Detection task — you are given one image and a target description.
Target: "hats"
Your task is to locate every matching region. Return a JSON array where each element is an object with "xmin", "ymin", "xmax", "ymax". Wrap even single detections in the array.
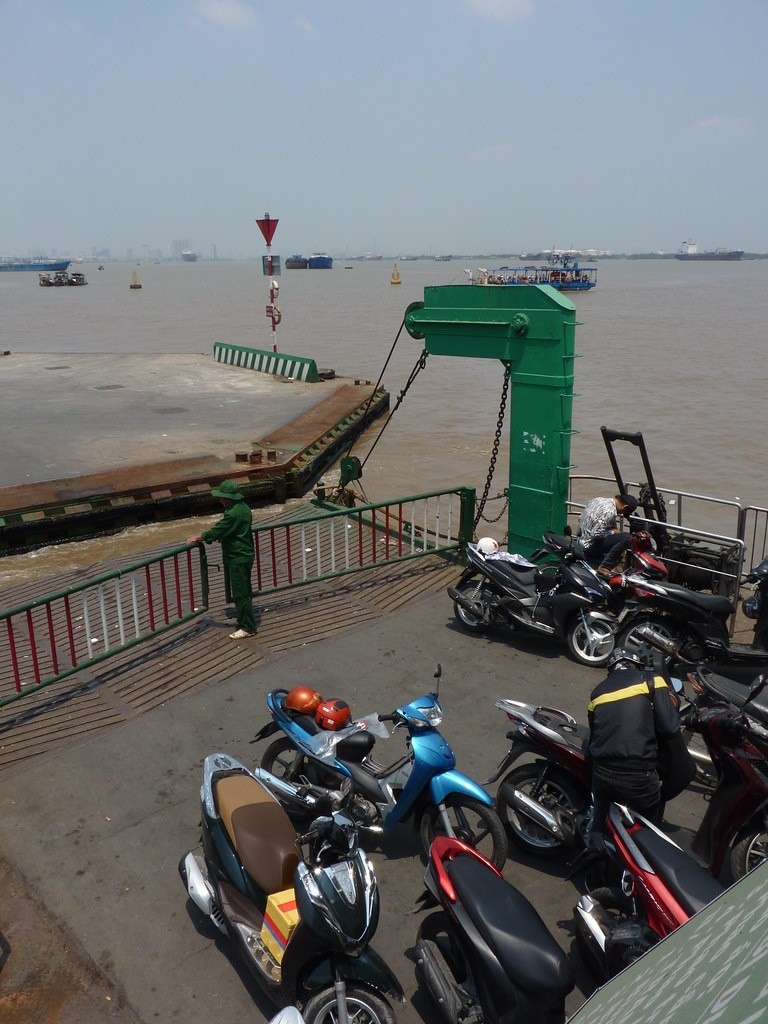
[
  {"xmin": 622, "ymin": 495, "xmax": 638, "ymax": 519},
  {"xmin": 210, "ymin": 480, "xmax": 244, "ymax": 500}
]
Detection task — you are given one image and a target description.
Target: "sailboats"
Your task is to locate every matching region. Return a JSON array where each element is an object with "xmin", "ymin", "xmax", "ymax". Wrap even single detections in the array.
[{"xmin": 129, "ymin": 270, "xmax": 142, "ymax": 289}]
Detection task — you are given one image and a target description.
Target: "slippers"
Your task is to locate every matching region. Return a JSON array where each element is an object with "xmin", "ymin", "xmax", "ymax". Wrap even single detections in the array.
[{"xmin": 596, "ymin": 571, "xmax": 617, "ymax": 580}]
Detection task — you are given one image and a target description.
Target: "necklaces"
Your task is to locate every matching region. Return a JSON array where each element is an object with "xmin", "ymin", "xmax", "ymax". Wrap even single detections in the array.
[{"xmin": 576, "ymin": 494, "xmax": 638, "ymax": 579}]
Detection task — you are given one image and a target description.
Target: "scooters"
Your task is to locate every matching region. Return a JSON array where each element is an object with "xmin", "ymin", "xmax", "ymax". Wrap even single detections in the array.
[
  {"xmin": 177, "ymin": 752, "xmax": 407, "ymax": 1024},
  {"xmin": 404, "ymin": 835, "xmax": 662, "ymax": 1024},
  {"xmin": 563, "ymin": 800, "xmax": 727, "ymax": 991},
  {"xmin": 542, "ymin": 524, "xmax": 768, "ymax": 789},
  {"xmin": 478, "ymin": 671, "xmax": 768, "ymax": 885}
]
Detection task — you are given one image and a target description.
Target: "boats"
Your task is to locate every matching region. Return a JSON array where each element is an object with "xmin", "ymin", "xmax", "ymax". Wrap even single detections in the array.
[
  {"xmin": 180, "ymin": 246, "xmax": 199, "ymax": 263},
  {"xmin": 434, "ymin": 253, "xmax": 453, "ymax": 262},
  {"xmin": 308, "ymin": 251, "xmax": 334, "ymax": 270},
  {"xmin": 284, "ymin": 251, "xmax": 309, "ymax": 269},
  {"xmin": 0, "ymin": 258, "xmax": 72, "ymax": 273},
  {"xmin": 97, "ymin": 264, "xmax": 104, "ymax": 271},
  {"xmin": 674, "ymin": 236, "xmax": 744, "ymax": 262},
  {"xmin": 39, "ymin": 268, "xmax": 88, "ymax": 287},
  {"xmin": 461, "ymin": 240, "xmax": 600, "ymax": 291},
  {"xmin": 389, "ymin": 261, "xmax": 403, "ymax": 284}
]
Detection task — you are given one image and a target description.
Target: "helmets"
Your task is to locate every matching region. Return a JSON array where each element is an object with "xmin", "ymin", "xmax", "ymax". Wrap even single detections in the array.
[
  {"xmin": 476, "ymin": 537, "xmax": 499, "ymax": 555},
  {"xmin": 315, "ymin": 697, "xmax": 352, "ymax": 731},
  {"xmin": 281, "ymin": 683, "xmax": 323, "ymax": 717},
  {"xmin": 607, "ymin": 646, "xmax": 644, "ymax": 668}
]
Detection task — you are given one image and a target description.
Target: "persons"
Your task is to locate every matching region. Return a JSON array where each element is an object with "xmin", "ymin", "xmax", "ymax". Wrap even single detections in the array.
[
  {"xmin": 487, "ymin": 273, "xmax": 590, "ymax": 284},
  {"xmin": 587, "ymin": 660, "xmax": 682, "ymax": 839},
  {"xmin": 185, "ymin": 480, "xmax": 257, "ymax": 639}
]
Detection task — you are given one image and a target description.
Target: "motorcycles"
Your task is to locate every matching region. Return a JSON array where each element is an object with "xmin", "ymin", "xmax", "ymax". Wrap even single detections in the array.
[
  {"xmin": 249, "ymin": 664, "xmax": 509, "ymax": 874},
  {"xmin": 447, "ymin": 525, "xmax": 625, "ymax": 668}
]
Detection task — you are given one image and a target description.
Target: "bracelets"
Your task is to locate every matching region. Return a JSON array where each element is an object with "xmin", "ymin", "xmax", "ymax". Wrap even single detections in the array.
[{"xmin": 611, "ymin": 530, "xmax": 615, "ymax": 535}]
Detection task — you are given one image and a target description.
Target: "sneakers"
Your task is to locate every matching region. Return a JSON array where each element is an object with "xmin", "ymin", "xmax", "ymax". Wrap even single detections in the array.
[
  {"xmin": 222, "ymin": 618, "xmax": 240, "ymax": 627},
  {"xmin": 229, "ymin": 628, "xmax": 256, "ymax": 640}
]
